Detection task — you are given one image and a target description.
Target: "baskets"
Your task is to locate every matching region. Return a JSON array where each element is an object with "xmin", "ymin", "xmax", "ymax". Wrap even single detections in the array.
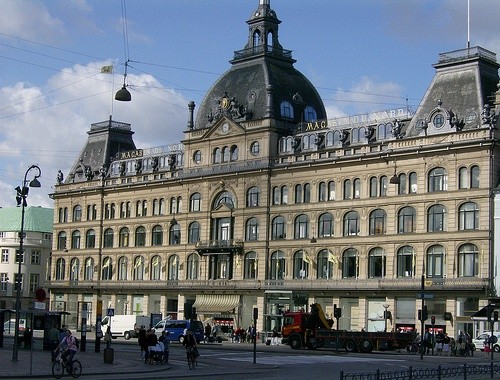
[{"xmin": 51, "ymin": 350, "xmax": 62, "ymax": 363}]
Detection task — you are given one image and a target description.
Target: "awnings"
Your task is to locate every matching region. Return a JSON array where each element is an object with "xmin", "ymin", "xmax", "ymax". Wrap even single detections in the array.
[
  {"xmin": 192, "ymin": 295, "xmax": 240, "ymax": 311},
  {"xmin": 471, "ymin": 303, "xmax": 500, "ymax": 321}
]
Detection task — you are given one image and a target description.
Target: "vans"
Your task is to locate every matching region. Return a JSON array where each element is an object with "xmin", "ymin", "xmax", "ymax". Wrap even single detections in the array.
[
  {"xmin": 471, "ymin": 332, "xmax": 500, "ymax": 352},
  {"xmin": 151, "ymin": 319, "xmax": 204, "ymax": 343},
  {"xmin": 4, "ymin": 318, "xmax": 26, "ymax": 331},
  {"xmin": 100, "ymin": 315, "xmax": 150, "ymax": 340}
]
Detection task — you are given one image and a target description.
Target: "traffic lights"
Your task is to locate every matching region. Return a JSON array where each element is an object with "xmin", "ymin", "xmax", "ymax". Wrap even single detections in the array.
[{"xmin": 431, "ymin": 316, "xmax": 435, "ymax": 324}]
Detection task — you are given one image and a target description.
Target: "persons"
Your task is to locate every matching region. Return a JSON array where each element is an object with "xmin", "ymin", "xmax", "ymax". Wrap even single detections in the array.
[
  {"xmin": 105, "ymin": 326, "xmax": 112, "ymax": 348},
  {"xmin": 272, "ymin": 326, "xmax": 278, "ymax": 345},
  {"xmin": 483, "ymin": 338, "xmax": 489, "ymax": 358},
  {"xmin": 183, "ymin": 330, "xmax": 199, "ymax": 366},
  {"xmin": 396, "ymin": 328, "xmax": 473, "ymax": 357},
  {"xmin": 57, "ymin": 330, "xmax": 77, "ymax": 374},
  {"xmin": 204, "ymin": 322, "xmax": 218, "ymax": 337},
  {"xmin": 138, "ymin": 325, "xmax": 169, "ymax": 364},
  {"xmin": 49, "ymin": 325, "xmax": 67, "ymax": 362},
  {"xmin": 231, "ymin": 326, "xmax": 254, "ymax": 343}
]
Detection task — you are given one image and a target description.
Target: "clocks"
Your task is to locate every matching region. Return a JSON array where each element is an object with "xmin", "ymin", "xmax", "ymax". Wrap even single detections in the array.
[{"xmin": 222, "ymin": 122, "xmax": 229, "ymax": 133}]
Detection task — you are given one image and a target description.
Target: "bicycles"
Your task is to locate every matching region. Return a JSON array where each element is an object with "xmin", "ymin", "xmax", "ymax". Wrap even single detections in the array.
[
  {"xmin": 463, "ymin": 343, "xmax": 474, "ymax": 357},
  {"xmin": 184, "ymin": 345, "xmax": 200, "ymax": 371},
  {"xmin": 51, "ymin": 347, "xmax": 83, "ymax": 380}
]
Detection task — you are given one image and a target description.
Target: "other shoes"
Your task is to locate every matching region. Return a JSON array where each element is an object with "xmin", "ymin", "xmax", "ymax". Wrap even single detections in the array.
[{"xmin": 70, "ymin": 367, "xmax": 73, "ymax": 372}]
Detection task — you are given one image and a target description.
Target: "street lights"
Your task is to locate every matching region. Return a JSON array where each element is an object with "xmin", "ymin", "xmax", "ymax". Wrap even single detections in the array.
[
  {"xmin": 12, "ymin": 165, "xmax": 41, "ymax": 361},
  {"xmin": 383, "ymin": 304, "xmax": 391, "ymax": 332}
]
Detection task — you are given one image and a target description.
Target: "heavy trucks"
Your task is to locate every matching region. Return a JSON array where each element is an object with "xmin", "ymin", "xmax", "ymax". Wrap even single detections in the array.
[{"xmin": 280, "ymin": 303, "xmax": 415, "ymax": 354}]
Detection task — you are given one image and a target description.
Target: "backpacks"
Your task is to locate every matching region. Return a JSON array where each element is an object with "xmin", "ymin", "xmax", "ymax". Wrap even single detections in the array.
[
  {"xmin": 70, "ymin": 336, "xmax": 79, "ymax": 349},
  {"xmin": 105, "ymin": 332, "xmax": 110, "ymax": 341}
]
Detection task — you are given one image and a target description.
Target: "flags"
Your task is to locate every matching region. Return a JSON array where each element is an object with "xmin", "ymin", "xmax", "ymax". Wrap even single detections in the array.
[
  {"xmin": 152, "ymin": 258, "xmax": 158, "ymax": 266},
  {"xmin": 303, "ymin": 253, "xmax": 314, "ymax": 268},
  {"xmin": 103, "ymin": 260, "xmax": 109, "ymax": 268},
  {"xmin": 134, "ymin": 257, "xmax": 141, "ymax": 268},
  {"xmin": 173, "ymin": 259, "xmax": 176, "ymax": 265},
  {"xmin": 72, "ymin": 259, "xmax": 90, "ymax": 272},
  {"xmin": 328, "ymin": 252, "xmax": 341, "ymax": 268},
  {"xmin": 236, "ymin": 256, "xmax": 279, "ymax": 269}
]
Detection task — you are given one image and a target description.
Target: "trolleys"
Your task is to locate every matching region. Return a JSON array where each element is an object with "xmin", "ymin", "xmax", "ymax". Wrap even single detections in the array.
[{"xmin": 148, "ymin": 344, "xmax": 169, "ymax": 366}]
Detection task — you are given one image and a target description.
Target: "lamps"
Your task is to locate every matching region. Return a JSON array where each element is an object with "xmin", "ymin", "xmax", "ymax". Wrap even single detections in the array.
[{"xmin": 114, "ymin": 61, "xmax": 131, "ymax": 102}]
